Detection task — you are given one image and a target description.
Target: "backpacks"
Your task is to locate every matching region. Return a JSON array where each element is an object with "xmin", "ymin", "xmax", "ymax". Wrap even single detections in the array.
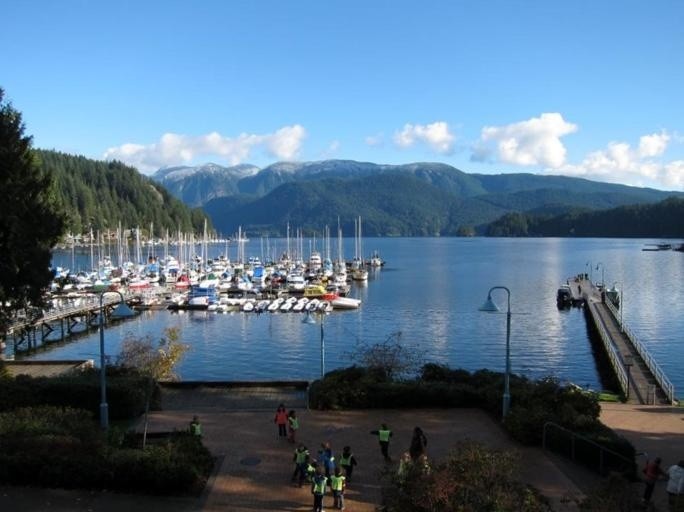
[
  {"xmin": 314, "ymin": 483, "xmax": 325, "ymax": 495},
  {"xmin": 293, "ymin": 421, "xmax": 298, "ymax": 429}
]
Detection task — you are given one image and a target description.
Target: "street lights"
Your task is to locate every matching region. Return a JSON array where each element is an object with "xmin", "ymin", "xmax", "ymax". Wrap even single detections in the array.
[
  {"xmin": 299, "ymin": 305, "xmax": 326, "ymax": 380},
  {"xmin": 585, "ymin": 260, "xmax": 593, "ymax": 281},
  {"xmin": 612, "ymin": 282, "xmax": 624, "ymax": 331},
  {"xmin": 596, "ymin": 260, "xmax": 606, "ymax": 300},
  {"xmin": 97, "ymin": 289, "xmax": 136, "ymax": 433},
  {"xmin": 477, "ymin": 285, "xmax": 513, "ymax": 424}
]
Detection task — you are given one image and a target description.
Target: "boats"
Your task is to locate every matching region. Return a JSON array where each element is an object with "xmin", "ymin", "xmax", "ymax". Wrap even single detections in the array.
[
  {"xmin": 596, "ymin": 280, "xmax": 619, "ymax": 305},
  {"xmin": 556, "ymin": 284, "xmax": 572, "ymax": 303},
  {"xmin": 657, "ymin": 242, "xmax": 671, "ymax": 250}
]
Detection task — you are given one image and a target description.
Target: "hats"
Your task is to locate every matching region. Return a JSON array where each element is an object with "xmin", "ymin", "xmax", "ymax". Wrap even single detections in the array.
[
  {"xmin": 400, "ymin": 448, "xmax": 408, "ymax": 453},
  {"xmin": 296, "ymin": 443, "xmax": 303, "ymax": 447}
]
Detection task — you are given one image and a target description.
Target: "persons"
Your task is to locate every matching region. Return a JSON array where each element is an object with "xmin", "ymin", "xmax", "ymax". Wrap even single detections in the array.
[
  {"xmin": 657, "ymin": 459, "xmax": 684, "ymax": 511},
  {"xmin": 291, "ymin": 441, "xmax": 357, "ymax": 488},
  {"xmin": 640, "ymin": 456, "xmax": 669, "ymax": 501},
  {"xmin": 409, "ymin": 427, "xmax": 427, "ymax": 461},
  {"xmin": 371, "ymin": 423, "xmax": 394, "ymax": 462},
  {"xmin": 188, "ymin": 415, "xmax": 200, "ymax": 437},
  {"xmin": 287, "ymin": 410, "xmax": 298, "ymax": 443},
  {"xmin": 311, "ymin": 466, "xmax": 328, "ymax": 512},
  {"xmin": 329, "ymin": 466, "xmax": 346, "ymax": 510},
  {"xmin": 274, "ymin": 404, "xmax": 289, "ymax": 439}
]
